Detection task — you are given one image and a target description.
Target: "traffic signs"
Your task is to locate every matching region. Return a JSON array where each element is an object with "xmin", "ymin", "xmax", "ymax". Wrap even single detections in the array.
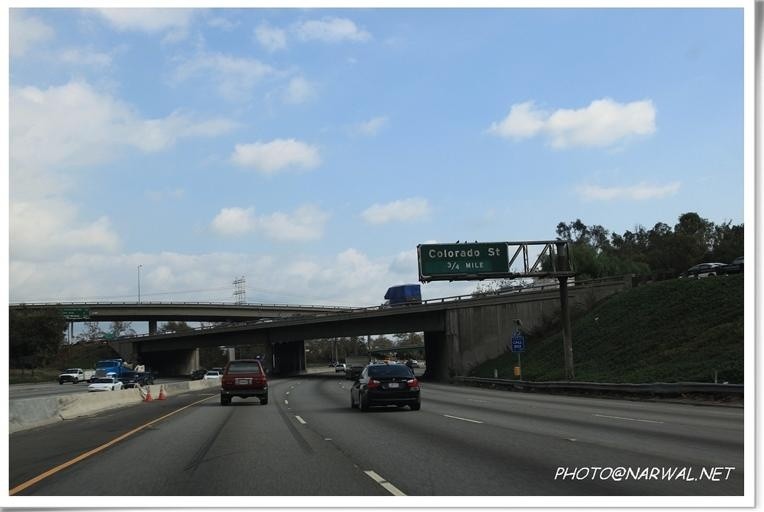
[{"xmin": 417, "ymin": 241, "xmax": 509, "ymax": 279}]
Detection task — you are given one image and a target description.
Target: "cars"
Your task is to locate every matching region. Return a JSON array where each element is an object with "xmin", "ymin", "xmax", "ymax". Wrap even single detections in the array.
[
  {"xmin": 328, "ymin": 357, "xmax": 424, "ymax": 378},
  {"xmin": 729, "ymin": 256, "xmax": 743, "ymax": 274},
  {"xmin": 348, "ymin": 361, "xmax": 422, "ymax": 410},
  {"xmin": 677, "ymin": 261, "xmax": 729, "ymax": 279},
  {"xmin": 58, "ymin": 357, "xmax": 224, "ymax": 391}
]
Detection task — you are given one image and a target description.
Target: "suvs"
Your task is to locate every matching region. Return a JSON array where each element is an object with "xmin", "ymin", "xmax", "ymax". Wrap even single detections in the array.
[{"xmin": 220, "ymin": 358, "xmax": 268, "ymax": 406}]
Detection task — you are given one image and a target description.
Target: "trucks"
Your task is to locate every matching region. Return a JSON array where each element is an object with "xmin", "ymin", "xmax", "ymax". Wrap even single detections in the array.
[{"xmin": 379, "ymin": 284, "xmax": 421, "ymax": 309}]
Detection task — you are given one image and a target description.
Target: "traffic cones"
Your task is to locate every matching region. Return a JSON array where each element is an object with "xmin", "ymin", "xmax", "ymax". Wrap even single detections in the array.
[
  {"xmin": 157, "ymin": 385, "xmax": 168, "ymax": 399},
  {"xmin": 143, "ymin": 385, "xmax": 154, "ymax": 401}
]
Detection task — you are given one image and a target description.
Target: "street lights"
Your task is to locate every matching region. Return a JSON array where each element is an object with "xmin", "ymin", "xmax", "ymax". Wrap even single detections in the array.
[{"xmin": 136, "ymin": 264, "xmax": 144, "ymax": 302}]
